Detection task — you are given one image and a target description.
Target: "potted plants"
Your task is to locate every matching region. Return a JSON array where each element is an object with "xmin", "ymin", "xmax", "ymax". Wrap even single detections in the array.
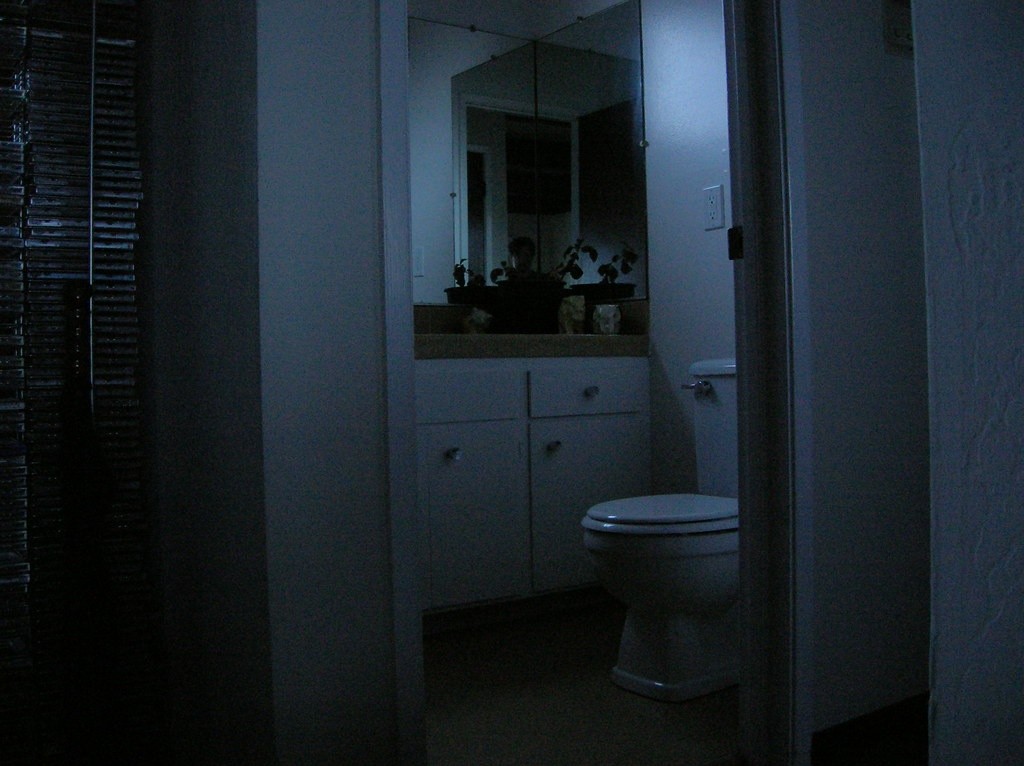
[
  {"xmin": 489, "ymin": 236, "xmax": 597, "ymax": 334},
  {"xmin": 570, "ymin": 240, "xmax": 641, "ymax": 299},
  {"xmin": 443, "ymin": 259, "xmax": 490, "ymax": 303}
]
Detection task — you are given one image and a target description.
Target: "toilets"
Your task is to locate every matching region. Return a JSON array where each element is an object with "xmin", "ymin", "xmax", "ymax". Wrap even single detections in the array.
[{"xmin": 580, "ymin": 355, "xmax": 744, "ymax": 705}]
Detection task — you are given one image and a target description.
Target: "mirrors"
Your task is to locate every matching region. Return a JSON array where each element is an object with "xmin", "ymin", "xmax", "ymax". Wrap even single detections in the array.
[{"xmin": 408, "ymin": 17, "xmax": 649, "ymax": 305}]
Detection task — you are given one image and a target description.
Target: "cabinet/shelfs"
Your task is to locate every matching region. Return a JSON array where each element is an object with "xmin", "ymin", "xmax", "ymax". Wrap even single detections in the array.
[{"xmin": 415, "ymin": 357, "xmax": 649, "ymax": 617}]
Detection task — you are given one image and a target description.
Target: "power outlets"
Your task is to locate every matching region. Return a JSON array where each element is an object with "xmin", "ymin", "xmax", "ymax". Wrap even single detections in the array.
[{"xmin": 701, "ymin": 184, "xmax": 725, "ymax": 231}]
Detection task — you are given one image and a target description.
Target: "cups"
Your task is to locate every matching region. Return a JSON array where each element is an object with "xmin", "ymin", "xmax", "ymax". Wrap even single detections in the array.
[{"xmin": 593, "ymin": 303, "xmax": 622, "ymax": 334}]
